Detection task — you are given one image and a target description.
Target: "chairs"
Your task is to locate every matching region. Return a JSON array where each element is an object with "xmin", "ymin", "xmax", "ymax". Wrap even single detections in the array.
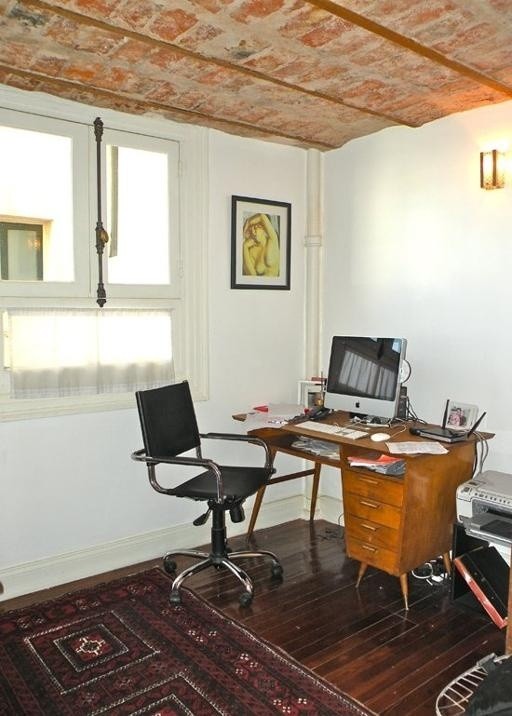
[{"xmin": 130, "ymin": 378, "xmax": 284, "ymax": 608}]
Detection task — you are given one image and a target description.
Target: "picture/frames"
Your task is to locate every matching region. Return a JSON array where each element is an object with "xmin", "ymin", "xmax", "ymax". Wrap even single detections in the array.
[{"xmin": 230, "ymin": 194, "xmax": 292, "ymax": 290}]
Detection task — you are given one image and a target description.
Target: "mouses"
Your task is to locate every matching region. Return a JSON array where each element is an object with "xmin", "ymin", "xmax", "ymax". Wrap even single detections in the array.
[{"xmin": 370, "ymin": 432, "xmax": 391, "ymax": 442}]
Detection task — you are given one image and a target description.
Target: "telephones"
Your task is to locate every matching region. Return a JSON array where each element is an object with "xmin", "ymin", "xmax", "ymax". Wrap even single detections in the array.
[{"xmin": 310, "ymin": 406, "xmax": 330, "ymax": 420}]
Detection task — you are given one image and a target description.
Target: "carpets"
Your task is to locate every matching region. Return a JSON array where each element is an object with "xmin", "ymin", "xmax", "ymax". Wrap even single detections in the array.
[{"xmin": 1, "ymin": 564, "xmax": 377, "ymax": 716}]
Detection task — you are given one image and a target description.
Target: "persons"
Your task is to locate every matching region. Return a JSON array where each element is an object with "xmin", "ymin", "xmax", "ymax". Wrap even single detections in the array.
[
  {"xmin": 447, "ymin": 406, "xmax": 467, "ymax": 427},
  {"xmin": 243, "ymin": 214, "xmax": 279, "ymax": 276}
]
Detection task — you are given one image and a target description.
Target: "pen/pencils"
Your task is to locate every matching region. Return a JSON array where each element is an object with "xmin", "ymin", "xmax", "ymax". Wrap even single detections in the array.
[{"xmin": 367, "ymin": 423, "xmax": 390, "ymax": 428}]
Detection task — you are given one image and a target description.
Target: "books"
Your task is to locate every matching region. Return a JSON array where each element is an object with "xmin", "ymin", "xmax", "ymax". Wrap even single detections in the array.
[
  {"xmin": 453, "ymin": 546, "xmax": 508, "ymax": 630},
  {"xmin": 292, "ymin": 435, "xmax": 450, "ymax": 475},
  {"xmin": 420, "ymin": 426, "xmax": 466, "ymax": 443},
  {"xmin": 461, "ymin": 546, "xmax": 509, "ymax": 620},
  {"xmin": 297, "ymin": 375, "xmax": 329, "ymax": 417}
]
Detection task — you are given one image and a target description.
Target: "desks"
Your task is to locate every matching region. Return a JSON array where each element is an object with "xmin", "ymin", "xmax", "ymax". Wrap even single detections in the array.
[{"xmin": 232, "ymin": 410, "xmax": 495, "ymax": 614}]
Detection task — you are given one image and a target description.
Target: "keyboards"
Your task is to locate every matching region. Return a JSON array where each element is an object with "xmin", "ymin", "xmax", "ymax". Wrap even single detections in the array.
[{"xmin": 292, "ymin": 420, "xmax": 369, "ymax": 441}]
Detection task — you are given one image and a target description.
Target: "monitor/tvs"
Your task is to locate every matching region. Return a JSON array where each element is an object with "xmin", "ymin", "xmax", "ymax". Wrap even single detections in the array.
[{"xmin": 322, "ymin": 336, "xmax": 407, "ymax": 426}]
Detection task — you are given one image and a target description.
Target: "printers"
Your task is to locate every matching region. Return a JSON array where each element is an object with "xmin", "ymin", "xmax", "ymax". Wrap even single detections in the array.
[{"xmin": 456, "ymin": 470, "xmax": 511, "ymax": 549}]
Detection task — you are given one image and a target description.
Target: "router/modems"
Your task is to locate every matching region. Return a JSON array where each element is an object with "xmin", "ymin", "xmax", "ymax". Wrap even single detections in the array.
[{"xmin": 410, "ymin": 400, "xmax": 487, "ymax": 444}]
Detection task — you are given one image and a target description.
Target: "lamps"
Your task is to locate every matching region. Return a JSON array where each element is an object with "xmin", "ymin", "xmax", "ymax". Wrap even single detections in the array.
[{"xmin": 479, "ymin": 148, "xmax": 506, "ymax": 191}]
